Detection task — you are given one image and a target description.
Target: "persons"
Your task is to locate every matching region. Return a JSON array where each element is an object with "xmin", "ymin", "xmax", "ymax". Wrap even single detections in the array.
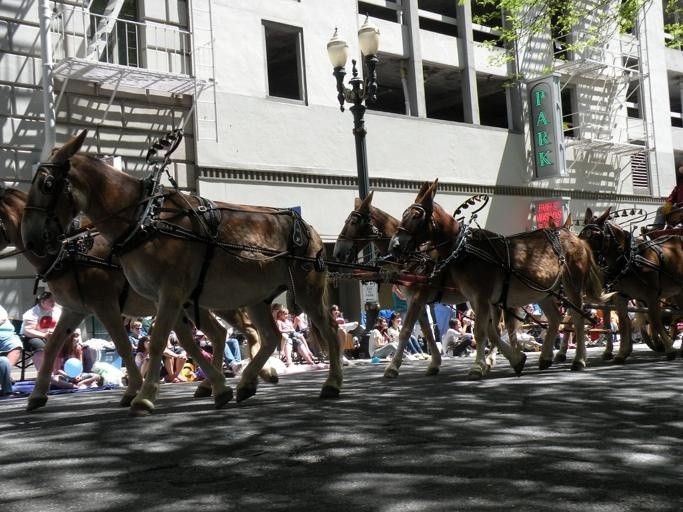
[
  {"xmin": 1, "ymin": 292, "xmax": 359, "ymax": 395},
  {"xmin": 645, "ymin": 179, "xmax": 683, "ymax": 240},
  {"xmin": 516, "ymin": 302, "xmax": 619, "ymax": 350},
  {"xmin": 360, "ymin": 301, "xmax": 476, "ymax": 362}
]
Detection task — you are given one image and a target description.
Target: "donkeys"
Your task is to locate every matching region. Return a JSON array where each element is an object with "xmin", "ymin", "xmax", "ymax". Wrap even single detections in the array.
[
  {"xmin": 386, "ymin": 179, "xmax": 600, "ymax": 380},
  {"xmin": 331, "ymin": 191, "xmax": 528, "ymax": 375},
  {"xmin": 540, "ymin": 209, "xmax": 682, "ymax": 363},
  {"xmin": 0, "ymin": 178, "xmax": 277, "ymax": 410},
  {"xmin": 21, "ymin": 128, "xmax": 344, "ymax": 417}
]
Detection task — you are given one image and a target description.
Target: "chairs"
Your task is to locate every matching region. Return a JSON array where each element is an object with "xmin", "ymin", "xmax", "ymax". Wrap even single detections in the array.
[{"xmin": 0, "ymin": 316, "xmax": 328, "ymax": 397}]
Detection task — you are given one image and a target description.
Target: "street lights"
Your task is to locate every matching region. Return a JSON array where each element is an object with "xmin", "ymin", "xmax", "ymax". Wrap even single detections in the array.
[{"xmin": 325, "ymin": 12, "xmax": 384, "ymax": 358}]
